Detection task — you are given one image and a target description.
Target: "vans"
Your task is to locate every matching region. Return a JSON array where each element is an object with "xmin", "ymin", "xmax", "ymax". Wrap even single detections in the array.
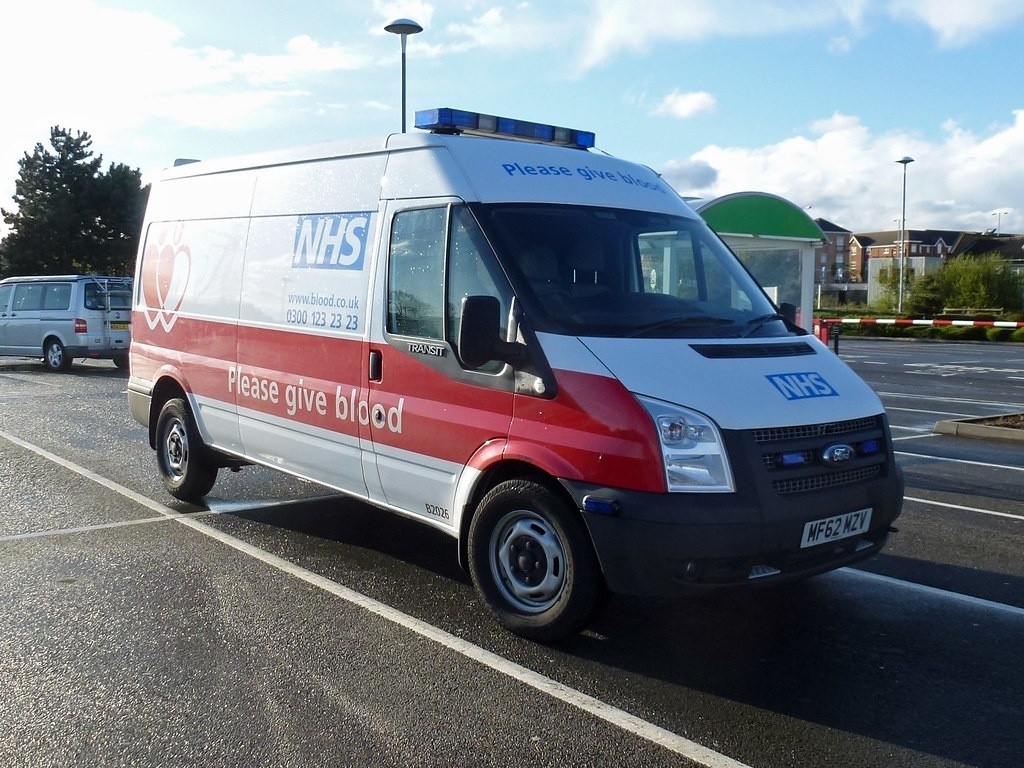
[
  {"xmin": 122, "ymin": 106, "xmax": 907, "ymax": 648},
  {"xmin": 0, "ymin": 276, "xmax": 135, "ymax": 374}
]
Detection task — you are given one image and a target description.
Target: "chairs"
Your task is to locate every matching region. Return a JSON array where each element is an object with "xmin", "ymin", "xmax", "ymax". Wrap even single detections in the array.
[{"xmin": 520, "ymin": 247, "xmax": 570, "ymax": 310}]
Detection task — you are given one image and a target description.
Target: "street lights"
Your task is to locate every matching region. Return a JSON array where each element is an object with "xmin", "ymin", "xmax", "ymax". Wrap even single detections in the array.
[{"xmin": 384, "ymin": 18, "xmax": 423, "ymax": 135}]
